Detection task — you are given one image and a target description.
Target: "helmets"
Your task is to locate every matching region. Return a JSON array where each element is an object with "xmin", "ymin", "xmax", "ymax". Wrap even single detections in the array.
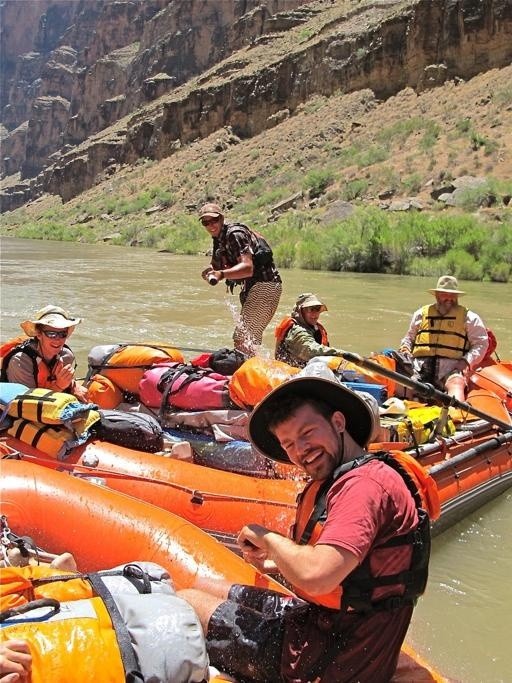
[{"xmin": 245, "ymin": 362, "xmax": 375, "ymax": 467}]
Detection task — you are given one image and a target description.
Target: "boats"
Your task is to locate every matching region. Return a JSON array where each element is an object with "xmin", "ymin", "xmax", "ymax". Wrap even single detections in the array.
[
  {"xmin": 0, "ymin": 449, "xmax": 442, "ymax": 682},
  {"xmin": 0, "ymin": 351, "xmax": 512, "ymax": 563}
]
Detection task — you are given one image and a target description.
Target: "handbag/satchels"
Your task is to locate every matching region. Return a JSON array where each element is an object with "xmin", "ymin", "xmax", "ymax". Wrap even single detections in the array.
[{"xmin": 84, "ymin": 341, "xmax": 301, "ymax": 453}]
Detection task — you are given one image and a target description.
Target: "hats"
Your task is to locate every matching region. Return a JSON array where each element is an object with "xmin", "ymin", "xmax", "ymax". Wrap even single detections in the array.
[
  {"xmin": 20, "ymin": 305, "xmax": 82, "ymax": 339},
  {"xmin": 426, "ymin": 276, "xmax": 467, "ymax": 298},
  {"xmin": 197, "ymin": 203, "xmax": 224, "ymax": 219},
  {"xmin": 297, "ymin": 293, "xmax": 324, "ymax": 309}
]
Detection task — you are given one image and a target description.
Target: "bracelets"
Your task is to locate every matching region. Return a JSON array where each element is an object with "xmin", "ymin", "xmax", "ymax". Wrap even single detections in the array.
[
  {"xmin": 218, "ymin": 269, "xmax": 225, "ymax": 280},
  {"xmin": 55, "ymin": 382, "xmax": 64, "ymax": 391}
]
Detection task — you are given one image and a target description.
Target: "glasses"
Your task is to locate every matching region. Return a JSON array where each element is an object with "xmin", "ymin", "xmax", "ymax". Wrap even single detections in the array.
[
  {"xmin": 201, "ymin": 216, "xmax": 220, "ymax": 226},
  {"xmin": 39, "ymin": 327, "xmax": 68, "ymax": 338},
  {"xmin": 303, "ymin": 306, "xmax": 321, "ymax": 313}
]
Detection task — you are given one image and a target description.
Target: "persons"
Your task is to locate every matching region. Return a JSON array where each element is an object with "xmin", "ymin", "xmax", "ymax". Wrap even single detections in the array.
[
  {"xmin": 398, "ymin": 275, "xmax": 490, "ymax": 402},
  {"xmin": 275, "ymin": 292, "xmax": 365, "ymax": 370},
  {"xmin": 176, "ymin": 361, "xmax": 432, "ymax": 682},
  {"xmin": 0, "ymin": 304, "xmax": 88, "ymax": 404},
  {"xmin": 0, "ymin": 638, "xmax": 34, "ymax": 683},
  {"xmin": 197, "ymin": 203, "xmax": 282, "ymax": 355}
]
